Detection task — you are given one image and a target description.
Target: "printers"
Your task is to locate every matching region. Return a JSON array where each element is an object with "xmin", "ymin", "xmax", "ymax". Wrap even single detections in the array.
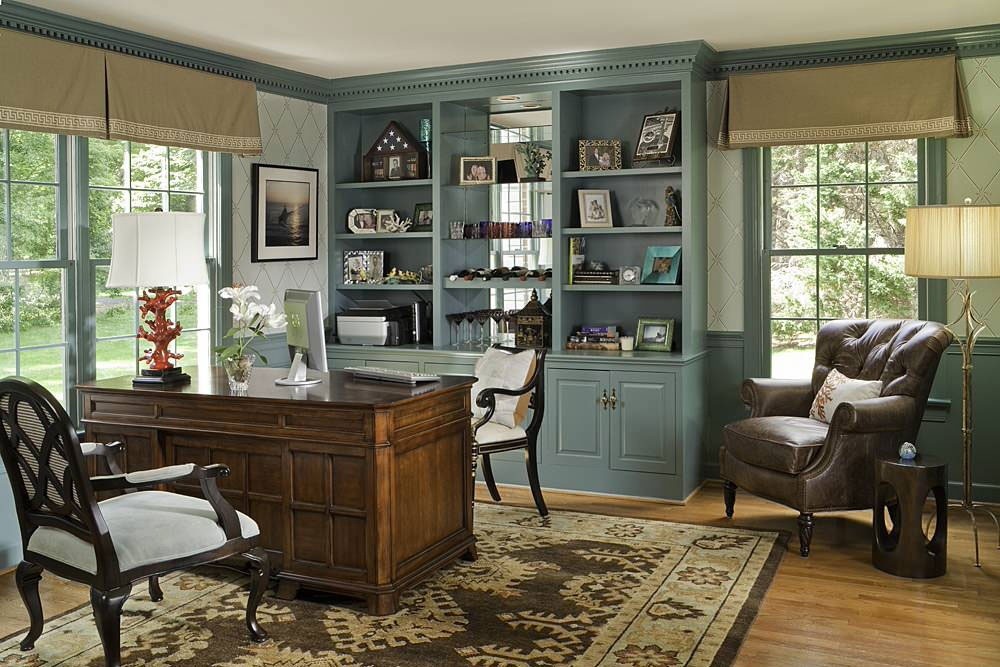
[{"xmin": 333, "ymin": 289, "xmax": 427, "ymax": 347}]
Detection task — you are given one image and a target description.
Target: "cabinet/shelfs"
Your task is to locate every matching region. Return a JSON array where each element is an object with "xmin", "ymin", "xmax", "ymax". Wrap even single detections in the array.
[{"xmin": 325, "ymin": 72, "xmax": 708, "ymax": 507}]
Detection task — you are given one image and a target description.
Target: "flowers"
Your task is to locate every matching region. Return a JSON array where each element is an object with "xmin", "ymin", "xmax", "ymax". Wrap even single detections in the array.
[{"xmin": 215, "ymin": 281, "xmax": 291, "ymax": 367}]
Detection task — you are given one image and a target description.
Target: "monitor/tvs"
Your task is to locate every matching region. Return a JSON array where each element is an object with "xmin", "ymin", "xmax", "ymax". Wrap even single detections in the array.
[{"xmin": 274, "ymin": 288, "xmax": 329, "ymax": 385}]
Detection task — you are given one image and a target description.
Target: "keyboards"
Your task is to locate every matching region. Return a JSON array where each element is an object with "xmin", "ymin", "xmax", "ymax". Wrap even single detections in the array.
[{"xmin": 344, "ymin": 365, "xmax": 441, "ymax": 384}]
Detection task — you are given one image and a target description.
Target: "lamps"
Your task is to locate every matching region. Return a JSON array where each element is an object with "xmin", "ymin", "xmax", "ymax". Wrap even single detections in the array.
[
  {"xmin": 103, "ymin": 208, "xmax": 210, "ymax": 387},
  {"xmin": 902, "ymin": 197, "xmax": 1000, "ymax": 569}
]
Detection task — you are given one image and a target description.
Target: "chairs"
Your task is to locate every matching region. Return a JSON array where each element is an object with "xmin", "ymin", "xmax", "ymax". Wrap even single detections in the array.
[
  {"xmin": 0, "ymin": 374, "xmax": 272, "ymax": 667},
  {"xmin": 720, "ymin": 318, "xmax": 953, "ymax": 555},
  {"xmin": 469, "ymin": 344, "xmax": 550, "ymax": 516}
]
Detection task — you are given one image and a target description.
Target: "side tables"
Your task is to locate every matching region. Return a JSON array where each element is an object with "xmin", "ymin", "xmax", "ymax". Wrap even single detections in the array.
[{"xmin": 872, "ymin": 457, "xmax": 950, "ymax": 578}]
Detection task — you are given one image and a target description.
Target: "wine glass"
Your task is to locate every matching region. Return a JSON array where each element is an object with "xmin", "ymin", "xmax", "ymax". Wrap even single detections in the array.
[{"xmin": 446, "ymin": 309, "xmax": 518, "ymax": 348}]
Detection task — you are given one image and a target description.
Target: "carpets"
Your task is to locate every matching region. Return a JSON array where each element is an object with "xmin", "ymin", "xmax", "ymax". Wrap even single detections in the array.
[{"xmin": 0, "ymin": 499, "xmax": 793, "ymax": 667}]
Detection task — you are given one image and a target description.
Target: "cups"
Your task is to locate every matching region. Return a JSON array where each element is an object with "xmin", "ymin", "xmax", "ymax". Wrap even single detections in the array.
[{"xmin": 450, "ymin": 219, "xmax": 552, "ymax": 239}]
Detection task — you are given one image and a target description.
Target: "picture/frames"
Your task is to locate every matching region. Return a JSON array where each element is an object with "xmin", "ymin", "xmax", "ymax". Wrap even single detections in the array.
[
  {"xmin": 632, "ymin": 111, "xmax": 680, "ymax": 161},
  {"xmin": 576, "ymin": 189, "xmax": 613, "ymax": 228},
  {"xmin": 458, "ymin": 156, "xmax": 498, "ymax": 185},
  {"xmin": 639, "ymin": 245, "xmax": 682, "ymax": 284},
  {"xmin": 411, "ymin": 203, "xmax": 433, "ymax": 230},
  {"xmin": 346, "ymin": 209, "xmax": 400, "ymax": 234},
  {"xmin": 369, "ymin": 250, "xmax": 385, "ymax": 280},
  {"xmin": 579, "ymin": 139, "xmax": 622, "ymax": 170},
  {"xmin": 375, "ymin": 208, "xmax": 395, "ymax": 232},
  {"xmin": 249, "ymin": 162, "xmax": 318, "ymax": 263},
  {"xmin": 342, "ymin": 249, "xmax": 370, "ymax": 283},
  {"xmin": 633, "ymin": 318, "xmax": 675, "ymax": 351}
]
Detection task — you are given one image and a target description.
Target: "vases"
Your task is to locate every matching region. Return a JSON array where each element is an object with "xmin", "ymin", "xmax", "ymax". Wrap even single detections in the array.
[{"xmin": 222, "ymin": 356, "xmax": 254, "ymax": 391}]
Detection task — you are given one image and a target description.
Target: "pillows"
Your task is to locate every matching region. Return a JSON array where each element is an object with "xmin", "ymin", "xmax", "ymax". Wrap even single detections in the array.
[
  {"xmin": 469, "ymin": 348, "xmax": 539, "ymax": 427},
  {"xmin": 805, "ymin": 364, "xmax": 885, "ymax": 426}
]
